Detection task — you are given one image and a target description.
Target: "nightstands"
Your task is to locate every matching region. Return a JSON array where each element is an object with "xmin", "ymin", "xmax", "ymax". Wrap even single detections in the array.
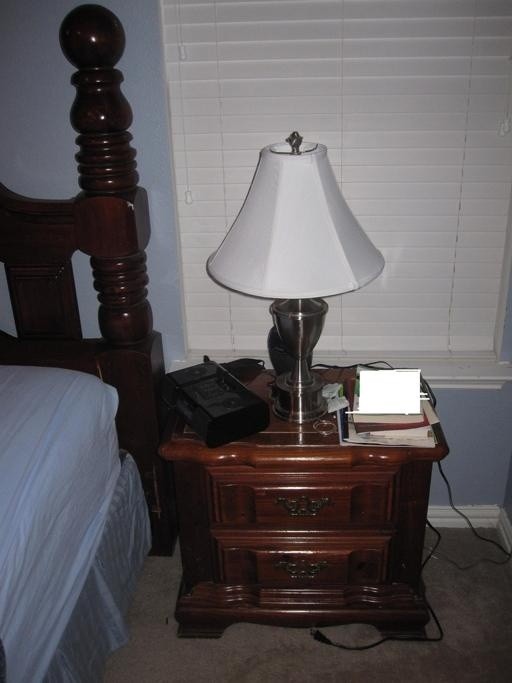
[{"xmin": 156, "ymin": 370, "xmax": 448, "ymax": 644}]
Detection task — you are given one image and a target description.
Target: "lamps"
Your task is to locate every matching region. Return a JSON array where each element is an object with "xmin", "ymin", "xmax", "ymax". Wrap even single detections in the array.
[{"xmin": 204, "ymin": 129, "xmax": 386, "ymax": 424}]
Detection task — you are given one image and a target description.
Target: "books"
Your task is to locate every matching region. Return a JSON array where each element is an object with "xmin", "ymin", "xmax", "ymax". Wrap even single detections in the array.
[{"xmin": 334, "ymin": 363, "xmax": 442, "ymax": 451}]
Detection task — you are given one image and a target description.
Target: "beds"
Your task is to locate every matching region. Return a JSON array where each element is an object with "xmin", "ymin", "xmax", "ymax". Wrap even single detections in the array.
[{"xmin": 1, "ymin": 4, "xmax": 180, "ymax": 683}]
